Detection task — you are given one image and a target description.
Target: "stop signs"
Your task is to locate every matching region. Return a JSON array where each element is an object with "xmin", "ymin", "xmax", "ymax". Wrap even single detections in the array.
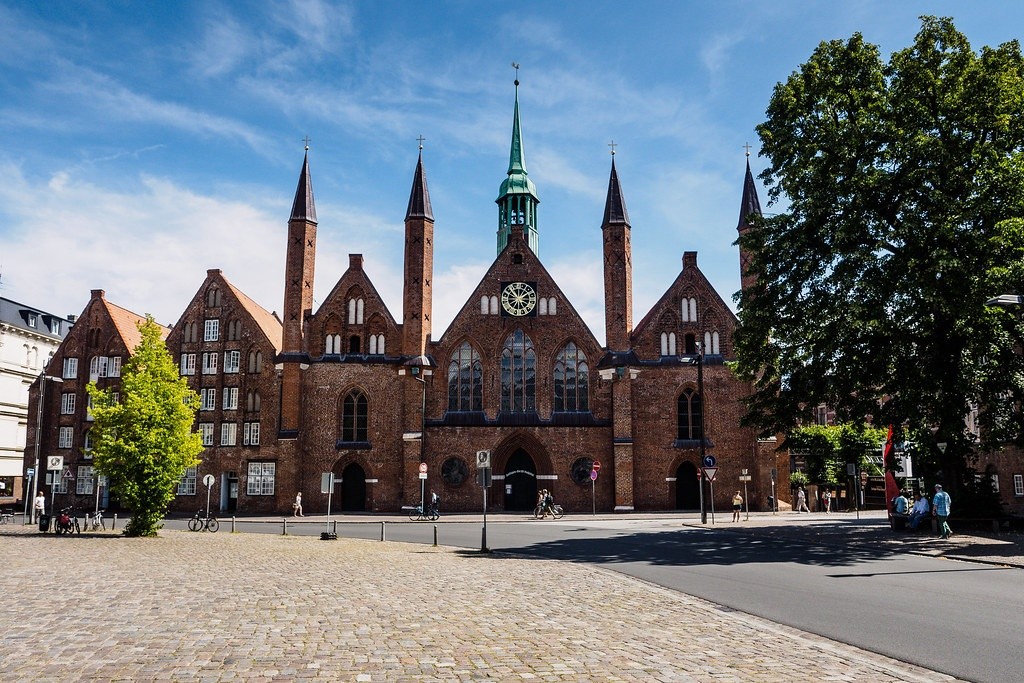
[
  {"xmin": 696, "ymin": 468, "xmax": 701, "ymax": 480},
  {"xmin": 593, "ymin": 461, "xmax": 601, "ymax": 472}
]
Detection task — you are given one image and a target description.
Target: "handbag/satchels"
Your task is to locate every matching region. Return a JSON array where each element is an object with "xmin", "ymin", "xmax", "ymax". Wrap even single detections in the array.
[{"xmin": 292, "ymin": 503, "xmax": 298, "ymax": 508}]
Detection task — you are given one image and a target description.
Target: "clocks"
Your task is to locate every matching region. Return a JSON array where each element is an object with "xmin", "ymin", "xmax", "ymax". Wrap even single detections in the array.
[{"xmin": 501, "ymin": 282, "xmax": 537, "ymax": 317}]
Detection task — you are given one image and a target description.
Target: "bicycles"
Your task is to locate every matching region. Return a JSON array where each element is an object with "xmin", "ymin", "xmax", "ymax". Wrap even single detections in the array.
[
  {"xmin": 535, "ymin": 499, "xmax": 564, "ymax": 520},
  {"xmin": 409, "ymin": 500, "xmax": 440, "ymax": 522},
  {"xmin": 187, "ymin": 509, "xmax": 220, "ymax": 534},
  {"xmin": 70, "ymin": 504, "xmax": 81, "ymax": 535},
  {"xmin": 92, "ymin": 510, "xmax": 106, "ymax": 532},
  {"xmin": 54, "ymin": 505, "xmax": 75, "ymax": 534}
]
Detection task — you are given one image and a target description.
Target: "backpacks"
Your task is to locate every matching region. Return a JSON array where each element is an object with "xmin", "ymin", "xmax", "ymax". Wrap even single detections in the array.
[
  {"xmin": 436, "ymin": 495, "xmax": 441, "ymax": 503},
  {"xmin": 544, "ymin": 495, "xmax": 552, "ymax": 506}
]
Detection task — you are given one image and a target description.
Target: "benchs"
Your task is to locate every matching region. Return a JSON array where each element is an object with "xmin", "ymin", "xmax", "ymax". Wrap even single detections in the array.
[{"xmin": 889, "ymin": 513, "xmax": 999, "ymax": 535}]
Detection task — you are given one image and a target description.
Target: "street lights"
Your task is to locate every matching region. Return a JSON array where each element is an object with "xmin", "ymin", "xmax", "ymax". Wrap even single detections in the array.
[
  {"xmin": 31, "ymin": 370, "xmax": 63, "ymax": 524},
  {"xmin": 681, "ymin": 354, "xmax": 708, "ymax": 525}
]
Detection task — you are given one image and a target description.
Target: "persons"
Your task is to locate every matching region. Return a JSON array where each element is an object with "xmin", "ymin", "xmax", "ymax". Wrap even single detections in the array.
[
  {"xmin": 797, "ymin": 487, "xmax": 810, "ymax": 514},
  {"xmin": 35, "ymin": 491, "xmax": 45, "ymax": 524},
  {"xmin": 430, "ymin": 488, "xmax": 440, "ymax": 520},
  {"xmin": 932, "ymin": 484, "xmax": 953, "ymax": 538},
  {"xmin": 891, "ymin": 491, "xmax": 909, "ymax": 514},
  {"xmin": 541, "ymin": 489, "xmax": 558, "ymax": 519},
  {"xmin": 822, "ymin": 488, "xmax": 832, "ymax": 514},
  {"xmin": 294, "ymin": 491, "xmax": 305, "ymax": 517},
  {"xmin": 732, "ymin": 490, "xmax": 743, "ymax": 522},
  {"xmin": 906, "ymin": 492, "xmax": 930, "ymax": 530},
  {"xmin": 534, "ymin": 490, "xmax": 543, "ymax": 518}
]
